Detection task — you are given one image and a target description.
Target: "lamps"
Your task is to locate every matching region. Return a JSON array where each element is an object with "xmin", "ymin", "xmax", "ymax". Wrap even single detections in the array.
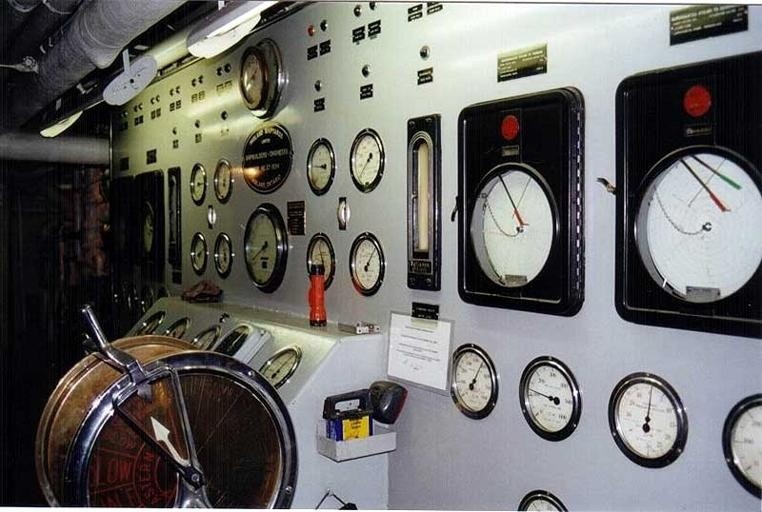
[
  {"xmin": 186, "ymin": 0, "xmax": 279, "ymax": 59},
  {"xmin": 20, "ymin": 82, "xmax": 105, "ymax": 138},
  {"xmin": 102, "ymin": 49, "xmax": 158, "ymax": 106}
]
